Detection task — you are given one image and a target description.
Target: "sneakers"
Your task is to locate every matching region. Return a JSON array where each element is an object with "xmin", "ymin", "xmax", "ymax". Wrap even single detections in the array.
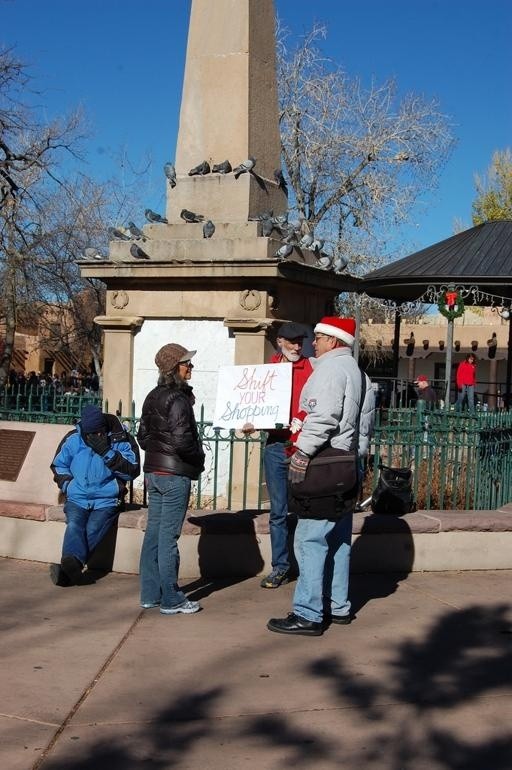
[
  {"xmin": 140, "ymin": 602, "xmax": 161, "ymax": 608},
  {"xmin": 51, "ymin": 564, "xmax": 68, "ymax": 585},
  {"xmin": 260, "ymin": 570, "xmax": 288, "ymax": 588},
  {"xmin": 159, "ymin": 600, "xmax": 200, "ymax": 613},
  {"xmin": 61, "ymin": 556, "xmax": 89, "ymax": 586}
]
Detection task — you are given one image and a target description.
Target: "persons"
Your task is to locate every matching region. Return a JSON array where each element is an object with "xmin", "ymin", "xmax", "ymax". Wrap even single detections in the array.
[
  {"xmin": 243, "ymin": 323, "xmax": 319, "ymax": 589},
  {"xmin": 414, "ymin": 375, "xmax": 439, "ymax": 460},
  {"xmin": 267, "ymin": 316, "xmax": 375, "ymax": 637},
  {"xmin": 48, "ymin": 404, "xmax": 139, "ymax": 586},
  {"xmin": 5, "ymin": 363, "xmax": 98, "ymax": 403},
  {"xmin": 455, "ymin": 353, "xmax": 477, "ymax": 415},
  {"xmin": 136, "ymin": 344, "xmax": 206, "ymax": 615}
]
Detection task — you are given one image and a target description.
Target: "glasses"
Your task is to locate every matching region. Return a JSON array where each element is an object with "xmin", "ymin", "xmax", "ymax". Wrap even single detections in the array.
[{"xmin": 178, "ymin": 360, "xmax": 191, "ymax": 367}]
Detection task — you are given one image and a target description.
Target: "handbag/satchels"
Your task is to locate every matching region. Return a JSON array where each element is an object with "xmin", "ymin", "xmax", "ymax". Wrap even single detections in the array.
[
  {"xmin": 287, "ymin": 448, "xmax": 358, "ymax": 519},
  {"xmin": 371, "ymin": 465, "xmax": 412, "ymax": 516}
]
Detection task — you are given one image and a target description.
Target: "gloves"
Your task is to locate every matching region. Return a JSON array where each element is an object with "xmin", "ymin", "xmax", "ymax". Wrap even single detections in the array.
[
  {"xmin": 282, "ymin": 451, "xmax": 310, "ymax": 484},
  {"xmin": 85, "ymin": 431, "xmax": 110, "ymax": 456}
]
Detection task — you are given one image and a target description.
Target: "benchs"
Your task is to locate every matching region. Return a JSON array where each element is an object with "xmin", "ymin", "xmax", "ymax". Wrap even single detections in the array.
[{"xmin": 0, "ymin": 501, "xmax": 512, "ymax": 581}]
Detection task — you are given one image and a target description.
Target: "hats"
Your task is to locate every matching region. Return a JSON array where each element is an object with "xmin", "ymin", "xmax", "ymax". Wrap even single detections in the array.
[
  {"xmin": 277, "ymin": 323, "xmax": 308, "ymax": 340},
  {"xmin": 81, "ymin": 406, "xmax": 105, "ymax": 433},
  {"xmin": 155, "ymin": 344, "xmax": 197, "ymax": 371},
  {"xmin": 414, "ymin": 375, "xmax": 428, "ymax": 383},
  {"xmin": 314, "ymin": 317, "xmax": 356, "ymax": 347}
]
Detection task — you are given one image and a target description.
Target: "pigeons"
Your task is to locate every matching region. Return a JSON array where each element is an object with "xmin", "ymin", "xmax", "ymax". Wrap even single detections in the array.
[
  {"xmin": 189, "ymin": 157, "xmax": 259, "ymax": 176},
  {"xmin": 130, "ymin": 243, "xmax": 150, "ymax": 260},
  {"xmin": 312, "ymin": 253, "xmax": 335, "ymax": 272},
  {"xmin": 329, "ymin": 252, "xmax": 350, "ymax": 273},
  {"xmin": 255, "ymin": 207, "xmax": 326, "ymax": 259},
  {"xmin": 164, "ymin": 162, "xmax": 175, "ymax": 186},
  {"xmin": 145, "ymin": 209, "xmax": 168, "ymax": 224},
  {"xmin": 77, "ymin": 241, "xmax": 104, "ymax": 260},
  {"xmin": 180, "ymin": 209, "xmax": 204, "ymax": 223},
  {"xmin": 108, "ymin": 222, "xmax": 150, "ymax": 240},
  {"xmin": 273, "ymin": 167, "xmax": 289, "ymax": 186},
  {"xmin": 204, "ymin": 221, "xmax": 215, "ymax": 238}
]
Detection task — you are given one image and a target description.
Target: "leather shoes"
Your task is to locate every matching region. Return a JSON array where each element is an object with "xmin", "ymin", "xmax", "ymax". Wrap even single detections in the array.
[
  {"xmin": 331, "ymin": 614, "xmax": 351, "ymax": 624},
  {"xmin": 268, "ymin": 612, "xmax": 322, "ymax": 635}
]
